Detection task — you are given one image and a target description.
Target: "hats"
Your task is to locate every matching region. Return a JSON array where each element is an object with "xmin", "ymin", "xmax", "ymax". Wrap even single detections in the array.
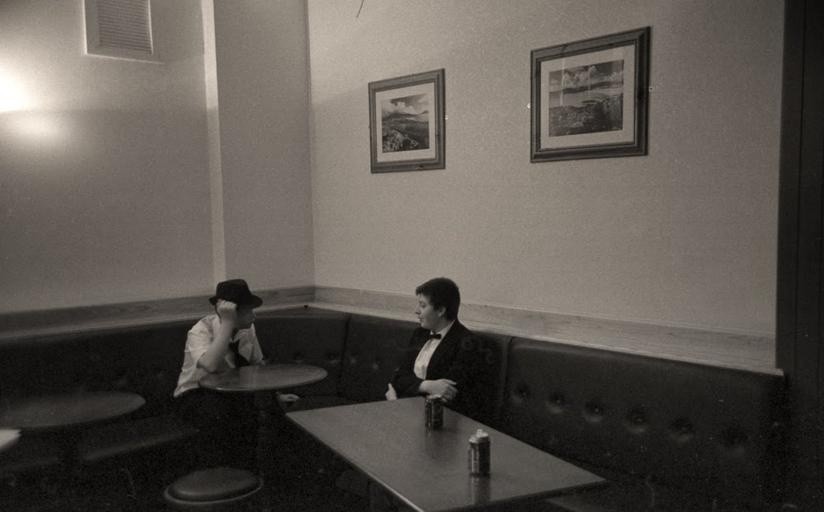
[{"xmin": 209, "ymin": 279, "xmax": 262, "ymax": 308}]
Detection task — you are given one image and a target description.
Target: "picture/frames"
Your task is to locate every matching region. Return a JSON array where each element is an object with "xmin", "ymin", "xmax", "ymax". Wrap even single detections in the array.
[
  {"xmin": 527, "ymin": 23, "xmax": 652, "ymax": 165},
  {"xmin": 365, "ymin": 66, "xmax": 448, "ymax": 175}
]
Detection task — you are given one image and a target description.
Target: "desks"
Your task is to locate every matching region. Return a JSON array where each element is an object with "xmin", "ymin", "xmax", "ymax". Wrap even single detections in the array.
[
  {"xmin": 280, "ymin": 396, "xmax": 609, "ymax": 511},
  {"xmin": 197, "ymin": 360, "xmax": 329, "ymax": 451},
  {"xmin": 0, "ymin": 386, "xmax": 142, "ymax": 447}
]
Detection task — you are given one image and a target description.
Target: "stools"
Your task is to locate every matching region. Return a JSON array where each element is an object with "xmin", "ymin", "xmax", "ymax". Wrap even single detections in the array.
[{"xmin": 160, "ymin": 462, "xmax": 263, "ymax": 510}]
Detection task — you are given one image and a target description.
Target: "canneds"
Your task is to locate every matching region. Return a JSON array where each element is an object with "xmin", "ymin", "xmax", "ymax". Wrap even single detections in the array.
[
  {"xmin": 466, "ymin": 429, "xmax": 491, "ymax": 478},
  {"xmin": 424, "ymin": 394, "xmax": 443, "ymax": 430}
]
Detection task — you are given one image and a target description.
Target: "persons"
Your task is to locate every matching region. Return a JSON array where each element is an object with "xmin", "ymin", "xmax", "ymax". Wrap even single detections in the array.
[
  {"xmin": 378, "ymin": 277, "xmax": 488, "ymax": 423},
  {"xmin": 172, "ymin": 278, "xmax": 299, "ymax": 469}
]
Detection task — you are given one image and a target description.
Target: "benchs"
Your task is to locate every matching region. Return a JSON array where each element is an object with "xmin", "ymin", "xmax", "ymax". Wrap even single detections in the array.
[
  {"xmin": 0, "ymin": 305, "xmax": 795, "ymax": 511},
  {"xmin": 6, "ymin": 406, "xmax": 188, "ymax": 487}
]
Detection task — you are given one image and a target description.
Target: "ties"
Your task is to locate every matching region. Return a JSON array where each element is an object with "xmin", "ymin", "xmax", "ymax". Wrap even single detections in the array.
[{"xmin": 229, "ymin": 340, "xmax": 248, "ymax": 366}]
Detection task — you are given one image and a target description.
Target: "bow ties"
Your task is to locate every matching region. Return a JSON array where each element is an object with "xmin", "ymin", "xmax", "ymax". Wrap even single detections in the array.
[{"xmin": 426, "ymin": 334, "xmax": 441, "ymax": 340}]
